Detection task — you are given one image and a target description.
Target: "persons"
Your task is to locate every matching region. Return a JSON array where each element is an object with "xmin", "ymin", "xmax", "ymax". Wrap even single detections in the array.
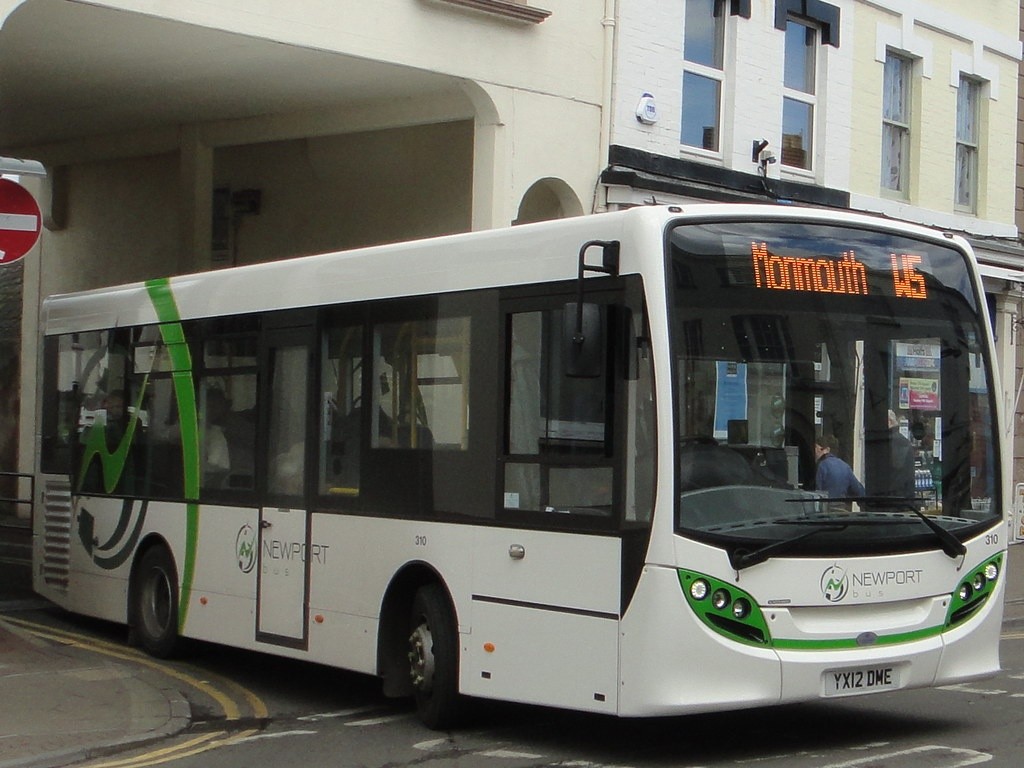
[
  {"xmin": 886, "ymin": 409, "xmax": 916, "ymax": 506},
  {"xmin": 812, "ymin": 434, "xmax": 867, "ymax": 514},
  {"xmin": 68, "ymin": 377, "xmax": 233, "ymax": 484}
]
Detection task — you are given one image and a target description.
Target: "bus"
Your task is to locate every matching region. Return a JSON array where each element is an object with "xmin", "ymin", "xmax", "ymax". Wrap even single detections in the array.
[{"xmin": 31, "ymin": 200, "xmax": 1010, "ymax": 733}]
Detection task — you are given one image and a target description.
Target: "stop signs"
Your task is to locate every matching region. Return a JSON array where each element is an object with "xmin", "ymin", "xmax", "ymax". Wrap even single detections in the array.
[{"xmin": 1, "ymin": 178, "xmax": 42, "ymax": 266}]
